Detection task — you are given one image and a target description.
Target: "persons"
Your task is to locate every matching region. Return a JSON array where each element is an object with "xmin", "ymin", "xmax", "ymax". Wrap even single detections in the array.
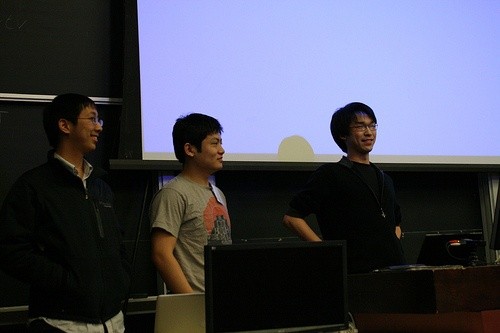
[
  {"xmin": 150, "ymin": 113, "xmax": 236, "ymax": 295},
  {"xmin": 282, "ymin": 102, "xmax": 412, "ymax": 273},
  {"xmin": 1, "ymin": 93, "xmax": 130, "ymax": 333}
]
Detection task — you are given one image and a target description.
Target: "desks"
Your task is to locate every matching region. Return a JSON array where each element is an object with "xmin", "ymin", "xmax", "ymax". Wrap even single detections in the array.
[{"xmin": 350, "ymin": 262, "xmax": 500, "ymax": 332}]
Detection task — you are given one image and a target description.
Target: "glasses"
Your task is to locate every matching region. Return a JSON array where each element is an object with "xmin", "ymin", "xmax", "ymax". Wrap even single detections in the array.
[
  {"xmin": 344, "ymin": 123, "xmax": 378, "ymax": 130},
  {"xmin": 74, "ymin": 116, "xmax": 103, "ymax": 127}
]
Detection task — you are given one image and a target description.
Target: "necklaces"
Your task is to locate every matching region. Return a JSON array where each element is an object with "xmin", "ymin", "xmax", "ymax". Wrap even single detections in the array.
[{"xmin": 342, "ymin": 167, "xmax": 386, "ymax": 219}]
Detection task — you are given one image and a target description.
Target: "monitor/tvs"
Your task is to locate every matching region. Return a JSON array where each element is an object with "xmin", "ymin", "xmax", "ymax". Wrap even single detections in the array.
[
  {"xmin": 203, "ymin": 240, "xmax": 350, "ymax": 333},
  {"xmin": 417, "ymin": 232, "xmax": 483, "ymax": 265}
]
